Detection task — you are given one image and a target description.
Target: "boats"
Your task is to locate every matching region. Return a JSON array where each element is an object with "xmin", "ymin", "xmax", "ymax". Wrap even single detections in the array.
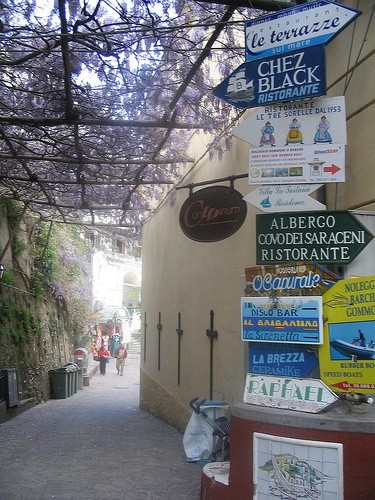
[{"xmin": 329, "ymin": 338, "xmax": 375, "ymax": 359}]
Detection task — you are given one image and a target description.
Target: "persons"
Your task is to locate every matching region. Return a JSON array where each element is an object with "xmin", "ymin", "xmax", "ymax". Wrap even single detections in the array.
[
  {"xmin": 114, "ymin": 344, "xmax": 127, "ymax": 376},
  {"xmin": 110, "ymin": 336, "xmax": 116, "ymax": 357},
  {"xmin": 99, "ymin": 346, "xmax": 109, "ymax": 376}
]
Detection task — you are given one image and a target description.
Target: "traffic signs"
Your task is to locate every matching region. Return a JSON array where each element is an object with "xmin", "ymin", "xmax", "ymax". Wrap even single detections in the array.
[
  {"xmin": 246, "ymin": 341, "xmax": 320, "ymax": 379},
  {"xmin": 240, "ymin": 372, "xmax": 341, "ymax": 410},
  {"xmin": 231, "ymin": 94, "xmax": 347, "ymax": 145},
  {"xmin": 240, "ymin": 298, "xmax": 324, "ymax": 347},
  {"xmin": 210, "ymin": 44, "xmax": 326, "ymax": 112},
  {"xmin": 241, "ymin": 184, "xmax": 328, "ymax": 213},
  {"xmin": 242, "ymin": 4, "xmax": 365, "ymax": 64},
  {"xmin": 319, "ymin": 274, "xmax": 375, "ymax": 393},
  {"xmin": 255, "ymin": 210, "xmax": 375, "ymax": 266},
  {"xmin": 243, "ymin": 260, "xmax": 342, "ymax": 297},
  {"xmin": 248, "ymin": 147, "xmax": 345, "ymax": 184}
]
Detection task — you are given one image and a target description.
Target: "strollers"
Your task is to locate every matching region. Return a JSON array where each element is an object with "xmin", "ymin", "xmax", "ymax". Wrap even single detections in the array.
[{"xmin": 190, "ymin": 395, "xmax": 231, "ymax": 464}]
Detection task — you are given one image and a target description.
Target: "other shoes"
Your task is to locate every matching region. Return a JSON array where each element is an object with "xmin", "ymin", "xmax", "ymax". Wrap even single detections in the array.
[
  {"xmin": 117, "ymin": 371, "xmax": 120, "ymax": 375},
  {"xmin": 120, "ymin": 372, "xmax": 123, "ymax": 376}
]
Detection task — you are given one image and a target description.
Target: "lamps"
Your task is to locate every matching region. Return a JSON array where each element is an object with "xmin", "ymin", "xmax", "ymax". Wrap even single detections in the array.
[{"xmin": 0, "ymin": 264, "xmax": 5, "ymax": 278}]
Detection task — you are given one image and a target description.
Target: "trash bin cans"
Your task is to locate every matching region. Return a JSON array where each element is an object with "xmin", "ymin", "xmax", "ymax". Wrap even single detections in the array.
[
  {"xmin": 50, "ymin": 363, "xmax": 84, "ymax": 399},
  {"xmin": 72, "ymin": 347, "xmax": 88, "ymax": 367}
]
